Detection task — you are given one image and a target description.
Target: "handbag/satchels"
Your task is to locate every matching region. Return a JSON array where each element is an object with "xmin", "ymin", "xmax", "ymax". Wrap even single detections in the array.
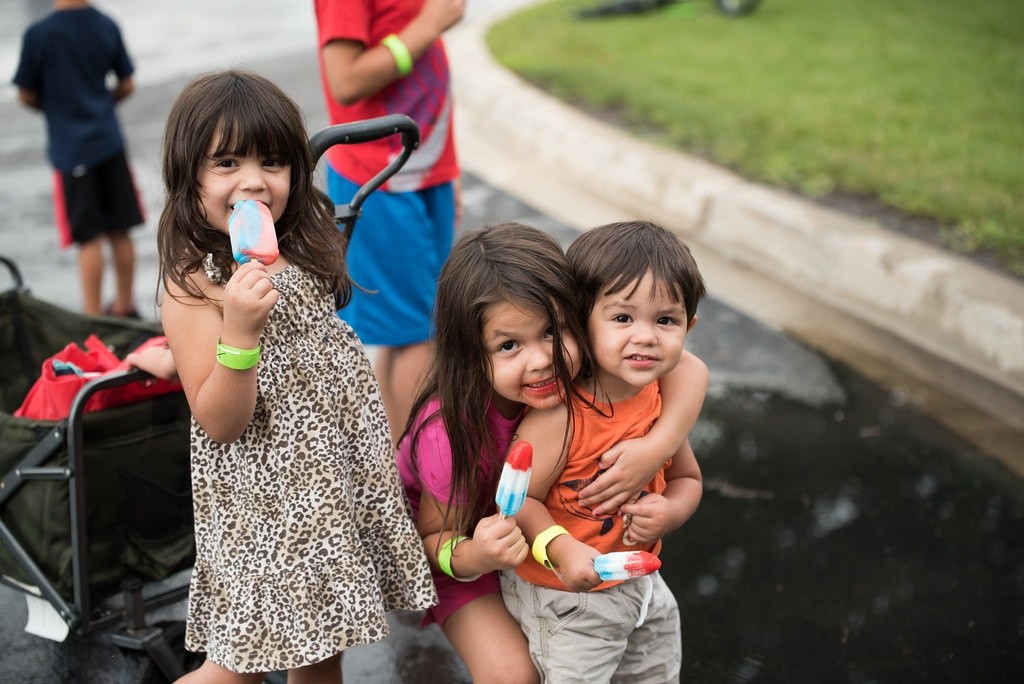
[{"xmin": 14, "ymin": 334, "xmax": 182, "ymax": 420}]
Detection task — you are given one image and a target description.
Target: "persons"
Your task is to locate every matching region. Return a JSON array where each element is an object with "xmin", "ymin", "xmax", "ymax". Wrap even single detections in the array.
[
  {"xmin": 312, "ymin": 0, "xmax": 470, "ymax": 441},
  {"xmin": 391, "ymin": 221, "xmax": 709, "ymax": 684},
  {"xmin": 155, "ymin": 71, "xmax": 440, "ymax": 684},
  {"xmin": 13, "ymin": 0, "xmax": 144, "ymax": 318},
  {"xmin": 500, "ymin": 220, "xmax": 703, "ymax": 684}
]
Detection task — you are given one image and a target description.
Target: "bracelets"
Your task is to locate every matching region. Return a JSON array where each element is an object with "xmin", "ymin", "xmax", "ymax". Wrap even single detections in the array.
[
  {"xmin": 438, "ymin": 536, "xmax": 482, "ymax": 582},
  {"xmin": 381, "ymin": 33, "xmax": 412, "ymax": 76},
  {"xmin": 215, "ymin": 335, "xmax": 261, "ymax": 370},
  {"xmin": 531, "ymin": 525, "xmax": 569, "ymax": 570}
]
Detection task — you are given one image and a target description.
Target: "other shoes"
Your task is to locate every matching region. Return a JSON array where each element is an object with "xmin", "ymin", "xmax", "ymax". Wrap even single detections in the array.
[{"xmin": 105, "ymin": 305, "xmax": 140, "ymax": 318}]
